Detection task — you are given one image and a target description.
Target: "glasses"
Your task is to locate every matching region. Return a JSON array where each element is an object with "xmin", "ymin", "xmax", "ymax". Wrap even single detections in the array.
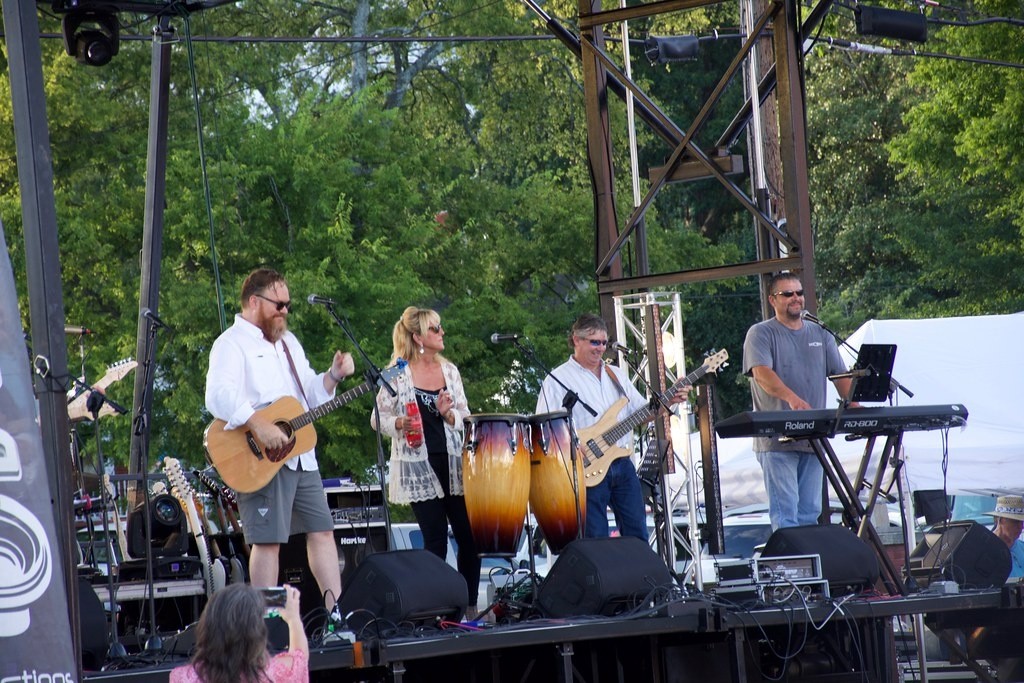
[
  {"xmin": 256, "ymin": 295, "xmax": 292, "ymax": 311},
  {"xmin": 429, "ymin": 326, "xmax": 440, "ymax": 334},
  {"xmin": 775, "ymin": 289, "xmax": 804, "ymax": 297},
  {"xmin": 584, "ymin": 338, "xmax": 608, "ymax": 346}
]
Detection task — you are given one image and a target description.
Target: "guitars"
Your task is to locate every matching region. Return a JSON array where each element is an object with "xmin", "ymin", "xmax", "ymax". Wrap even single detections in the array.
[
  {"xmin": 151, "ymin": 453, "xmax": 253, "ymax": 605},
  {"xmin": 65, "ymin": 356, "xmax": 139, "ymax": 423},
  {"xmin": 203, "ymin": 364, "xmax": 405, "ymax": 495},
  {"xmin": 573, "ymin": 347, "xmax": 730, "ymax": 488},
  {"xmin": 99, "ymin": 474, "xmax": 135, "ymax": 560}
]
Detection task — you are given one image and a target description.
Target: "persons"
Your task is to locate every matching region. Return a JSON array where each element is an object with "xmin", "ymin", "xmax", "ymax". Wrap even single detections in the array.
[
  {"xmin": 169, "ymin": 582, "xmax": 309, "ymax": 683},
  {"xmin": 741, "ymin": 273, "xmax": 863, "ymax": 533},
  {"xmin": 985, "ymin": 495, "xmax": 1024, "ymax": 683},
  {"xmin": 370, "ymin": 306, "xmax": 482, "ymax": 623},
  {"xmin": 205, "ymin": 270, "xmax": 356, "ymax": 616},
  {"xmin": 0, "ymin": 384, "xmax": 104, "ymax": 578},
  {"xmin": 535, "ymin": 313, "xmax": 694, "ymax": 545}
]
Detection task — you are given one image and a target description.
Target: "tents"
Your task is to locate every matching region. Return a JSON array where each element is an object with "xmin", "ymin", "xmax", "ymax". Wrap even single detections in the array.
[{"xmin": 667, "ymin": 310, "xmax": 1024, "ymax": 546}]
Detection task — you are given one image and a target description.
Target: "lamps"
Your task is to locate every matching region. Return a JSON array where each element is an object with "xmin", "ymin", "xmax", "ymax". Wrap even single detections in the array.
[
  {"xmin": 126, "ymin": 493, "xmax": 190, "ymax": 558},
  {"xmin": 49, "ymin": 0, "xmax": 121, "ymax": 66}
]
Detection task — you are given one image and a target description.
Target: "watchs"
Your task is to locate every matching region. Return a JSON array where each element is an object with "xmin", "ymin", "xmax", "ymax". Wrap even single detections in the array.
[{"xmin": 327, "ymin": 366, "xmax": 345, "ymax": 383}]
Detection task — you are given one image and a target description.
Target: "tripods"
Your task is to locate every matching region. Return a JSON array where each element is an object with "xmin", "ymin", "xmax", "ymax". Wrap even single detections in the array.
[{"xmin": 475, "ymin": 512, "xmax": 545, "ymax": 626}]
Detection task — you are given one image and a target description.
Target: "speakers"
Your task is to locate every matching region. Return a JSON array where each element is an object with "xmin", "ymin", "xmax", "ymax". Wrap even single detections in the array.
[
  {"xmin": 539, "ymin": 537, "xmax": 672, "ymax": 619},
  {"xmin": 901, "ymin": 521, "xmax": 1010, "ymax": 592},
  {"xmin": 78, "ymin": 580, "xmax": 112, "ymax": 668},
  {"xmin": 762, "ymin": 525, "xmax": 880, "ymax": 600},
  {"xmin": 340, "ymin": 549, "xmax": 468, "ymax": 635}
]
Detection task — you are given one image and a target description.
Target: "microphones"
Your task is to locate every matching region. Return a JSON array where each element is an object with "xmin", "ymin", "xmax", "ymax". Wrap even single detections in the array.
[
  {"xmin": 64, "ymin": 326, "xmax": 92, "ymax": 337},
  {"xmin": 308, "ymin": 294, "xmax": 335, "ymax": 305},
  {"xmin": 142, "ymin": 308, "xmax": 166, "ymax": 328},
  {"xmin": 799, "ymin": 310, "xmax": 825, "ymax": 327},
  {"xmin": 613, "ymin": 342, "xmax": 632, "ymax": 354},
  {"xmin": 491, "ymin": 333, "xmax": 526, "ymax": 343}
]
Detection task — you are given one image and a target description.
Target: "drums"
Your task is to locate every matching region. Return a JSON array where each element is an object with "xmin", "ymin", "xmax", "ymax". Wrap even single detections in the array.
[
  {"xmin": 528, "ymin": 409, "xmax": 587, "ymax": 556},
  {"xmin": 461, "ymin": 412, "xmax": 531, "ymax": 559}
]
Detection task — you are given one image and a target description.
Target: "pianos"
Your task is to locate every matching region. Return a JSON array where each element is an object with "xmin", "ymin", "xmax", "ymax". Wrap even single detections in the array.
[{"xmin": 714, "ymin": 402, "xmax": 970, "ymax": 598}]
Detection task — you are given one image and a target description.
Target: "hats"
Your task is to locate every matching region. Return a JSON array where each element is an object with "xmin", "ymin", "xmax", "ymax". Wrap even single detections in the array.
[{"xmin": 982, "ymin": 495, "xmax": 1024, "ymax": 523}]
